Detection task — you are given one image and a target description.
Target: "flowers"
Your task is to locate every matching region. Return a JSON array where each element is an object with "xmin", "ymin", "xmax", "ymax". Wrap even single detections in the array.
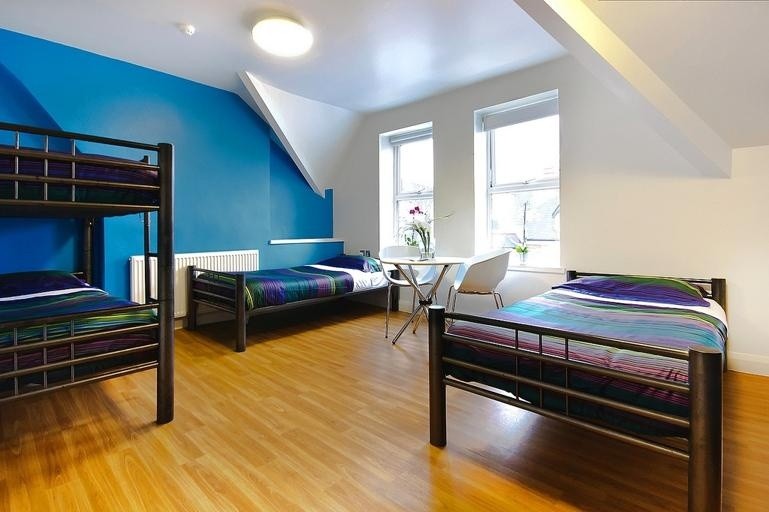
[{"xmin": 401, "ymin": 206, "xmax": 431, "ymax": 258}]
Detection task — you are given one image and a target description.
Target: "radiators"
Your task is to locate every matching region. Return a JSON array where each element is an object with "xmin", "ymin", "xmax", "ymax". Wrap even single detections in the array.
[{"xmin": 128, "ymin": 249, "xmax": 260, "ymax": 319}]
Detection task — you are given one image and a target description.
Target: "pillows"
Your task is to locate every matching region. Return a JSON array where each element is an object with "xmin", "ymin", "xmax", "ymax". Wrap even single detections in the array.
[
  {"xmin": 0, "ymin": 271, "xmax": 104, "ymax": 302},
  {"xmin": 550, "ymin": 274, "xmax": 709, "ymax": 307},
  {"xmin": 317, "ymin": 254, "xmax": 381, "ymax": 273}
]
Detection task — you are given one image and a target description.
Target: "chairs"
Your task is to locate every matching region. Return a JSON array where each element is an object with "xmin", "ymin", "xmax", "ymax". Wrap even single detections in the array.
[
  {"xmin": 378, "ymin": 245, "xmax": 439, "ymax": 337},
  {"xmin": 445, "ymin": 248, "xmax": 512, "ymax": 328}
]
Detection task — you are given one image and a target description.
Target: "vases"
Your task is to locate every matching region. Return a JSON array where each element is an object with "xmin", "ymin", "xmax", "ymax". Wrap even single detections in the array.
[{"xmin": 418, "ymin": 249, "xmax": 435, "ymax": 261}]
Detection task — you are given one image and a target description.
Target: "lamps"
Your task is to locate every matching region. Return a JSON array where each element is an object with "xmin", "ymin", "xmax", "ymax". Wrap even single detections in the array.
[{"xmin": 251, "ymin": 14, "xmax": 314, "ymax": 59}]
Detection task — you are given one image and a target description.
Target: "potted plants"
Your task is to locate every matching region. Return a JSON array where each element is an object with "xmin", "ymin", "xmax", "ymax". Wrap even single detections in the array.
[{"xmin": 515, "ymin": 245, "xmax": 528, "ymax": 265}]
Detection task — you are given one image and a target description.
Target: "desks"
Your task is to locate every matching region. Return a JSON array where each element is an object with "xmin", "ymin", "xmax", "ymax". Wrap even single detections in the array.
[{"xmin": 380, "ymin": 257, "xmax": 461, "ymax": 344}]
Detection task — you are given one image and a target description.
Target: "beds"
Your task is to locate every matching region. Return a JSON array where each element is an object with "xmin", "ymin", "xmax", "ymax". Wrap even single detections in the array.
[
  {"xmin": 0, "ymin": 121, "xmax": 174, "ymax": 426},
  {"xmin": 184, "ymin": 253, "xmax": 398, "ymax": 353},
  {"xmin": 429, "ymin": 271, "xmax": 729, "ymax": 510}
]
